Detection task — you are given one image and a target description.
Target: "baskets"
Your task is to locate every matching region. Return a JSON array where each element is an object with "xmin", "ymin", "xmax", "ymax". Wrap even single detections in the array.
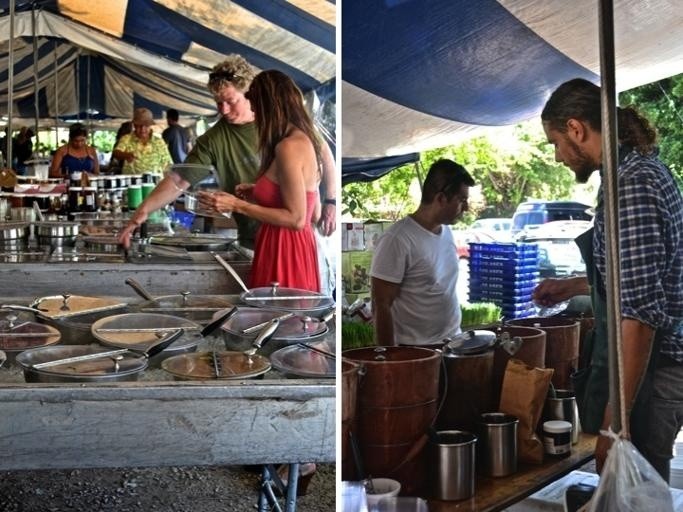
[
  {"xmin": 467, "ymin": 242, "xmax": 542, "ymax": 324},
  {"xmin": 171, "ymin": 212, "xmax": 195, "ymax": 228}
]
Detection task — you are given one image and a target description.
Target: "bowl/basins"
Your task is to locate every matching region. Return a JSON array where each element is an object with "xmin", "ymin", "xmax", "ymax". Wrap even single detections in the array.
[{"xmin": 185, "ymin": 192, "xmax": 232, "ymax": 219}]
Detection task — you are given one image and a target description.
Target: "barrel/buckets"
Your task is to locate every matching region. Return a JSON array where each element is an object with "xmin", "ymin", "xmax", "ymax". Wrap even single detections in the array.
[
  {"xmin": 570, "ymin": 312, "xmax": 597, "ymax": 353},
  {"xmin": 342, "ymin": 346, "xmax": 447, "ymax": 499},
  {"xmin": 475, "ymin": 325, "xmax": 547, "ymax": 413},
  {"xmin": 505, "ymin": 315, "xmax": 581, "ymax": 392},
  {"xmin": 440, "ymin": 346, "xmax": 496, "ymax": 433},
  {"xmin": 342, "ymin": 356, "xmax": 362, "ymax": 480}
]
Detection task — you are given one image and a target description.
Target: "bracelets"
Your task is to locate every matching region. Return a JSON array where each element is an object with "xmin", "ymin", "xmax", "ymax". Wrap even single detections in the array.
[
  {"xmin": 607, "ymin": 401, "xmax": 633, "ymax": 413},
  {"xmin": 322, "ymin": 198, "xmax": 338, "ymax": 205}
]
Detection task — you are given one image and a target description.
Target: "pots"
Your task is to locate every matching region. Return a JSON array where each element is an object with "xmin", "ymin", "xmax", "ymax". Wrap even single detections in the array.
[
  {"xmin": 0, "ymin": 208, "xmax": 254, "ymax": 250},
  {"xmin": 1, "ymin": 283, "xmax": 337, "ymax": 383}
]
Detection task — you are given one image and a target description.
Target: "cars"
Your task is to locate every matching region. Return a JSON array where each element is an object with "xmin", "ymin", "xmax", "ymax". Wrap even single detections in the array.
[{"xmin": 456, "ymin": 200, "xmax": 595, "ymax": 260}]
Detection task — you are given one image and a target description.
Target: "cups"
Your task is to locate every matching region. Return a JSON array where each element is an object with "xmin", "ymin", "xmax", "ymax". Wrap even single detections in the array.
[
  {"xmin": 479, "ymin": 411, "xmax": 519, "ymax": 479},
  {"xmin": 548, "ymin": 390, "xmax": 580, "ymax": 447},
  {"xmin": 363, "ymin": 478, "xmax": 401, "ymax": 512},
  {"xmin": 341, "ymin": 481, "xmax": 368, "ymax": 512},
  {"xmin": 434, "ymin": 431, "xmax": 476, "ymax": 500},
  {"xmin": 122, "ymin": 184, "xmax": 155, "ymax": 208},
  {"xmin": 544, "ymin": 419, "xmax": 572, "ymax": 458}
]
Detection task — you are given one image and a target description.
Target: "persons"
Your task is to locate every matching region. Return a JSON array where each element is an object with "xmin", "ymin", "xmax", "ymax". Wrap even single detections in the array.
[
  {"xmin": 0, "ymin": 107, "xmax": 192, "ymax": 175},
  {"xmin": 115, "ymin": 54, "xmax": 338, "ymax": 252},
  {"xmin": 533, "ymin": 78, "xmax": 683, "ymax": 512},
  {"xmin": 367, "ymin": 160, "xmax": 475, "ymax": 347},
  {"xmin": 196, "ymin": 69, "xmax": 324, "ymax": 496}
]
